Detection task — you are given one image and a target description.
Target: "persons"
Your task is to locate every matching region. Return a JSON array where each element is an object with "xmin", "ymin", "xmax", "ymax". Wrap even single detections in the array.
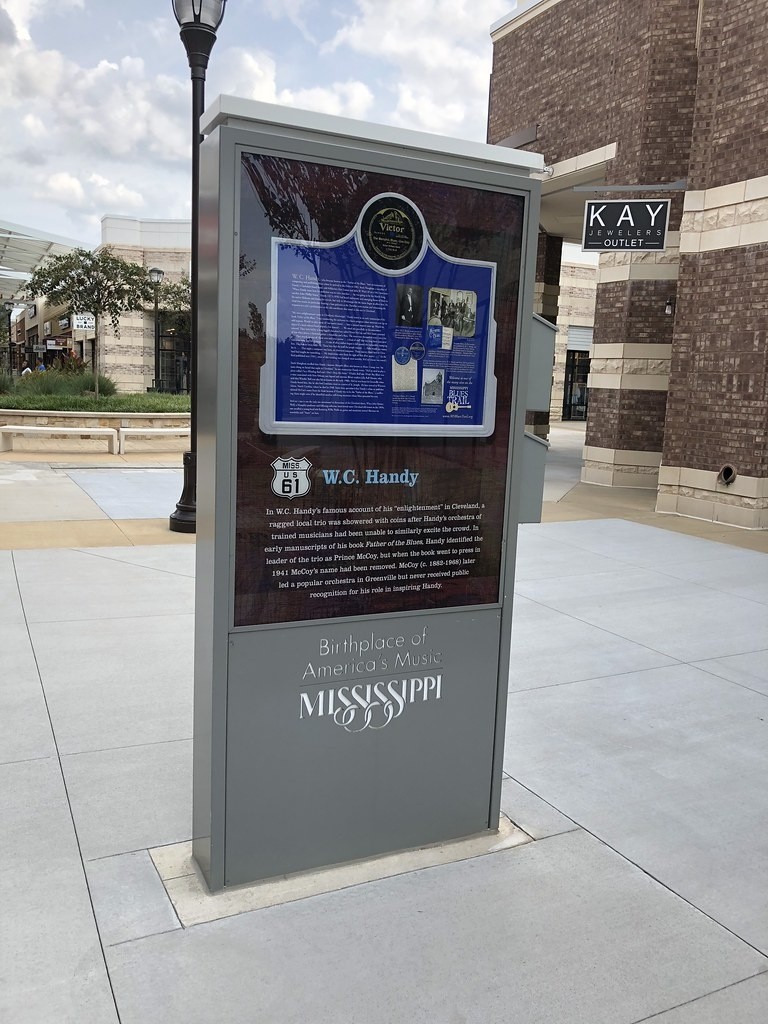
[
  {"xmin": 22, "ymin": 360, "xmax": 32, "ymax": 377},
  {"xmin": 401, "ymin": 288, "xmax": 416, "ymax": 326},
  {"xmin": 431, "ymin": 298, "xmax": 474, "ymax": 334},
  {"xmin": 36, "ymin": 357, "xmax": 45, "ymax": 371}
]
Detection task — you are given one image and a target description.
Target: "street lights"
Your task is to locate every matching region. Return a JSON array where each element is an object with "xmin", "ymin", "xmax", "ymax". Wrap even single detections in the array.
[{"xmin": 170, "ymin": 0, "xmax": 230, "ymax": 533}]
